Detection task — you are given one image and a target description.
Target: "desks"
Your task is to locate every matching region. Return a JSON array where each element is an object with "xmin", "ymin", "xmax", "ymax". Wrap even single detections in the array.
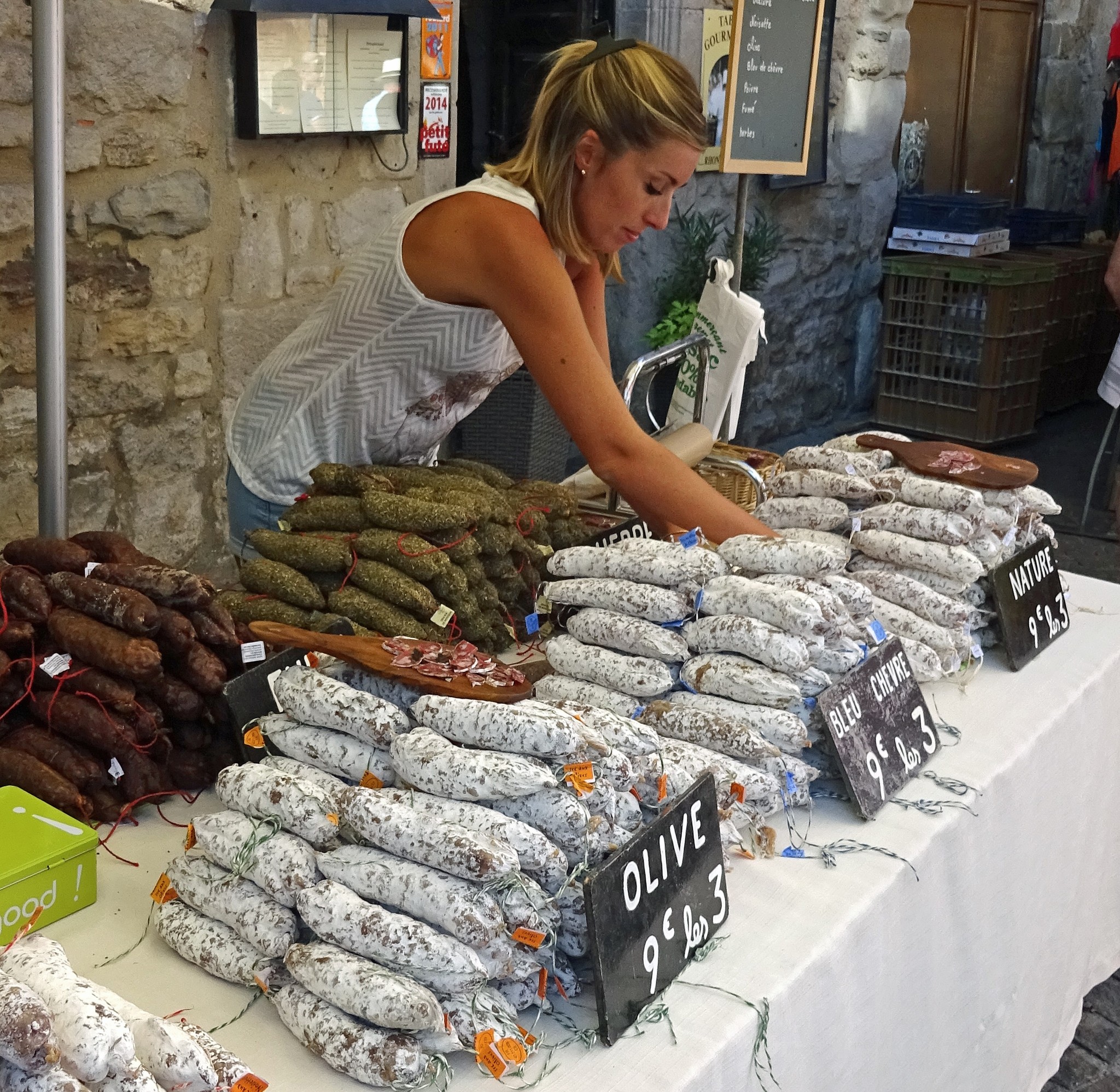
[{"xmin": 31, "ymin": 561, "xmax": 1120, "ymax": 1092}]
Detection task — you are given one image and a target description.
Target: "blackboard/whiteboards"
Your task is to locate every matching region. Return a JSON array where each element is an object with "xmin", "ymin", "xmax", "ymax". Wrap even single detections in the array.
[
  {"xmin": 582, "ymin": 771, "xmax": 732, "ymax": 1047},
  {"xmin": 816, "ymin": 637, "xmax": 944, "ymax": 822},
  {"xmin": 986, "ymin": 535, "xmax": 1073, "ymax": 673},
  {"xmin": 220, "ymin": 616, "xmax": 362, "ymax": 765},
  {"xmin": 719, "ymin": 0, "xmax": 825, "ymax": 177}
]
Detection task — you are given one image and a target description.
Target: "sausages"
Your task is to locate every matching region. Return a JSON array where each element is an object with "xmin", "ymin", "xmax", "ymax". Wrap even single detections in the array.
[
  {"xmin": 145, "ymin": 636, "xmax": 777, "ymax": 1092},
  {"xmin": 0, "ymin": 929, "xmax": 275, "ymax": 1092},
  {"xmin": 3, "ymin": 524, "xmax": 265, "ymax": 826},
  {"xmin": 535, "ymin": 426, "xmax": 1066, "ymax": 849},
  {"xmin": 225, "ymin": 456, "xmax": 617, "ymax": 651}
]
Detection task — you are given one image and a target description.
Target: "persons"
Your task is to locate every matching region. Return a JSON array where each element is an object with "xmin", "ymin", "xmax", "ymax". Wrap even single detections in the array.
[
  {"xmin": 263, "ymin": 52, "xmax": 402, "ymax": 130},
  {"xmin": 228, "ymin": 37, "xmax": 780, "ymax": 585}
]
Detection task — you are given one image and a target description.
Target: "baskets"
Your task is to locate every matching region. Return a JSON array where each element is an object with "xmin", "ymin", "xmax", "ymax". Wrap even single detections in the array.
[{"xmin": 696, "ymin": 441, "xmax": 783, "ymax": 514}]
